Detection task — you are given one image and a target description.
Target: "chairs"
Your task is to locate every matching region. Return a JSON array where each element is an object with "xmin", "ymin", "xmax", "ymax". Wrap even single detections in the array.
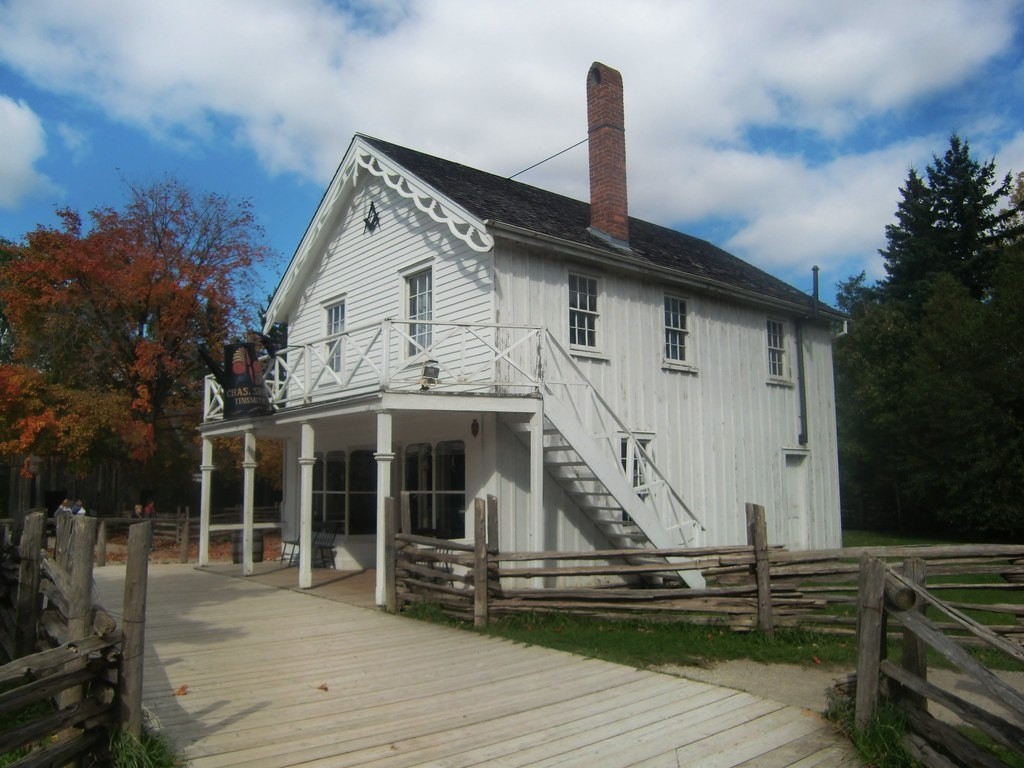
[{"xmin": 282, "ymin": 522, "xmax": 340, "ymax": 567}]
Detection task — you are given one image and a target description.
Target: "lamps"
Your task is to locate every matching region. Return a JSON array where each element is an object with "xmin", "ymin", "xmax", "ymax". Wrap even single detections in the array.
[{"xmin": 420, "ymin": 359, "xmax": 439, "ymax": 393}]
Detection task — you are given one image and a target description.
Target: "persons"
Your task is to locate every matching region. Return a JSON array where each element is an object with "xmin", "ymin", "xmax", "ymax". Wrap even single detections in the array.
[
  {"xmin": 134, "ymin": 500, "xmax": 159, "ymax": 551},
  {"xmin": 53, "ymin": 498, "xmax": 85, "ymax": 560}
]
